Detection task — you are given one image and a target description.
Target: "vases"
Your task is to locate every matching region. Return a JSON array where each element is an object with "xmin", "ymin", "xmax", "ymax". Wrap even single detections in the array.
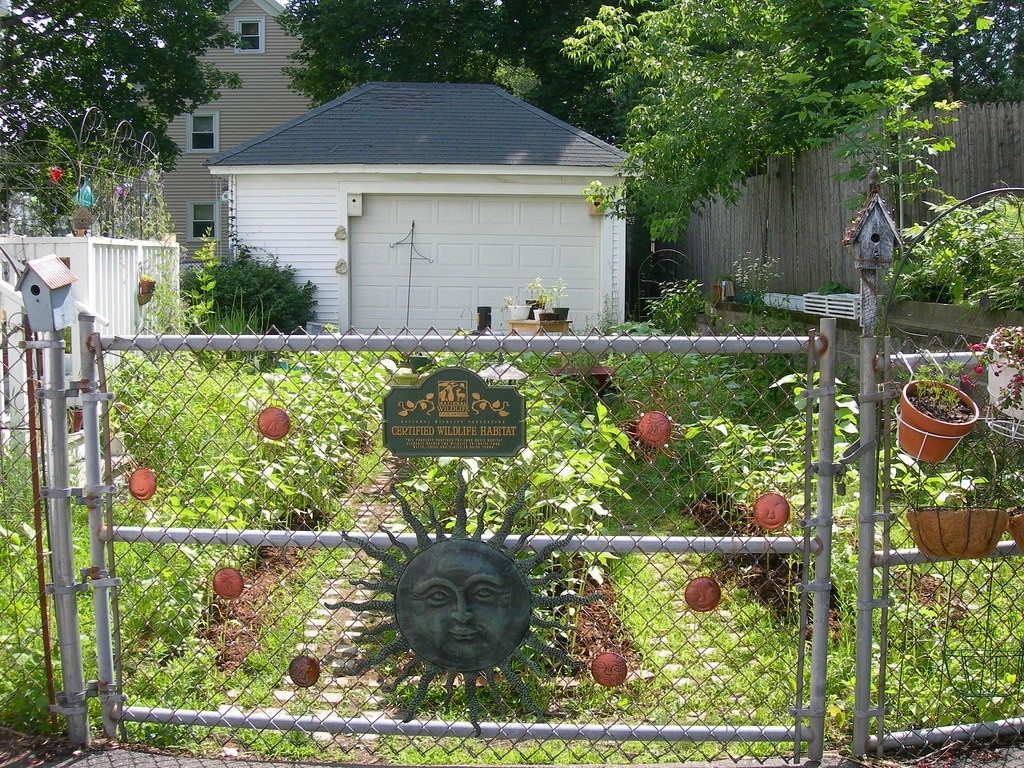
[
  {"xmin": 141, "ymin": 281, "xmax": 155, "ymax": 294},
  {"xmin": 525, "ymin": 300, "xmax": 545, "ymax": 320},
  {"xmin": 552, "ymin": 307, "xmax": 569, "ymax": 320},
  {"xmin": 532, "ymin": 309, "xmax": 545, "ymax": 320},
  {"xmin": 983, "ymin": 330, "xmax": 1024, "ymax": 420},
  {"xmin": 539, "ymin": 314, "xmax": 558, "ymax": 320},
  {"xmin": 507, "ymin": 306, "xmax": 531, "ymax": 321}
]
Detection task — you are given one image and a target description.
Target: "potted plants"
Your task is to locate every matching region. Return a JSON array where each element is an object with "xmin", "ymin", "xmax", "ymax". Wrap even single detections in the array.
[
  {"xmin": 899, "ymin": 360, "xmax": 978, "ymax": 461},
  {"xmin": 65, "ymin": 405, "xmax": 83, "ymax": 433},
  {"xmin": 907, "ymin": 495, "xmax": 1008, "ymax": 559},
  {"xmin": 1007, "ymin": 504, "xmax": 1024, "ymax": 556}
]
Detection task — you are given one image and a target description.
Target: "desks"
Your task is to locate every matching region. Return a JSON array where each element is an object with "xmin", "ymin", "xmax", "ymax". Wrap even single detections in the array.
[
  {"xmin": 509, "ymin": 321, "xmax": 573, "ymax": 337},
  {"xmin": 549, "ymin": 367, "xmax": 610, "ymax": 387}
]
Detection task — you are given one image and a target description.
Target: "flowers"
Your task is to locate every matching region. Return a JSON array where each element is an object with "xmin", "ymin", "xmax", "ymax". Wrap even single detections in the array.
[
  {"xmin": 141, "ymin": 273, "xmax": 154, "ymax": 282},
  {"xmin": 502, "ymin": 274, "xmax": 568, "ymax": 315},
  {"xmin": 964, "ymin": 325, "xmax": 1024, "ymax": 412}
]
